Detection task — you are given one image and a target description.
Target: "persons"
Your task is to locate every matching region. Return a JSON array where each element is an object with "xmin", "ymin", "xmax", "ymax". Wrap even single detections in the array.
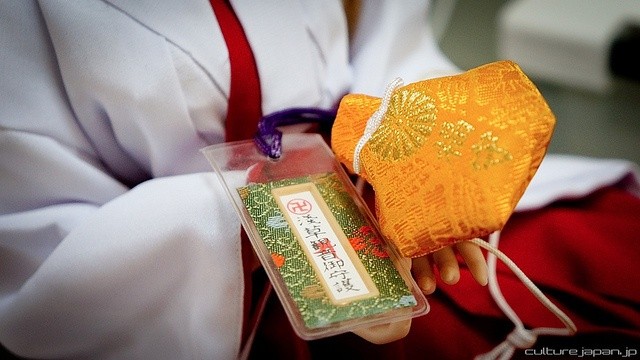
[{"xmin": 0, "ymin": 1, "xmax": 489, "ymax": 358}]
